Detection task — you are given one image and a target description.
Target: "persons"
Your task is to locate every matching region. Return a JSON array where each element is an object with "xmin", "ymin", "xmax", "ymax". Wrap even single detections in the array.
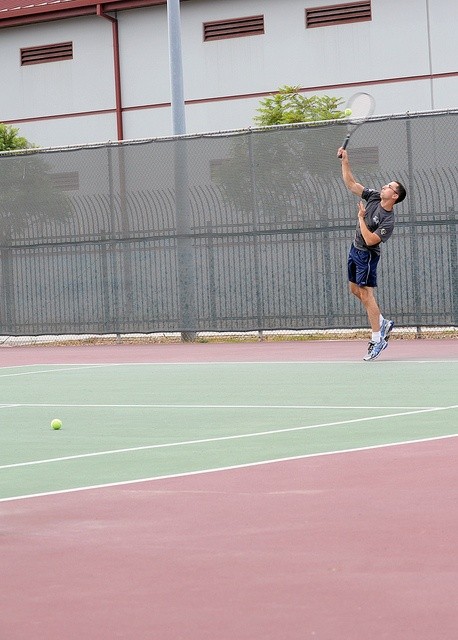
[{"xmin": 337, "ymin": 147, "xmax": 406, "ymax": 361}]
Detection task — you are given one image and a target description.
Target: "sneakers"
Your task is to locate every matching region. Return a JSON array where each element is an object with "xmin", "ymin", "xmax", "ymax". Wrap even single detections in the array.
[
  {"xmin": 363, "ymin": 337, "xmax": 388, "ymax": 361},
  {"xmin": 380, "ymin": 319, "xmax": 394, "ymax": 342}
]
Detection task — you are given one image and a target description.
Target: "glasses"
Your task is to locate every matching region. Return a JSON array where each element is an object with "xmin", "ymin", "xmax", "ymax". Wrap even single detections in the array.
[{"xmin": 386, "ymin": 184, "xmax": 397, "ymax": 194}]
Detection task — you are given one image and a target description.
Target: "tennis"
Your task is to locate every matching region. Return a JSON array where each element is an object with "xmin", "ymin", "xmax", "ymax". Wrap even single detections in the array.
[
  {"xmin": 345, "ymin": 109, "xmax": 352, "ymax": 116},
  {"xmin": 51, "ymin": 419, "xmax": 62, "ymax": 430}
]
